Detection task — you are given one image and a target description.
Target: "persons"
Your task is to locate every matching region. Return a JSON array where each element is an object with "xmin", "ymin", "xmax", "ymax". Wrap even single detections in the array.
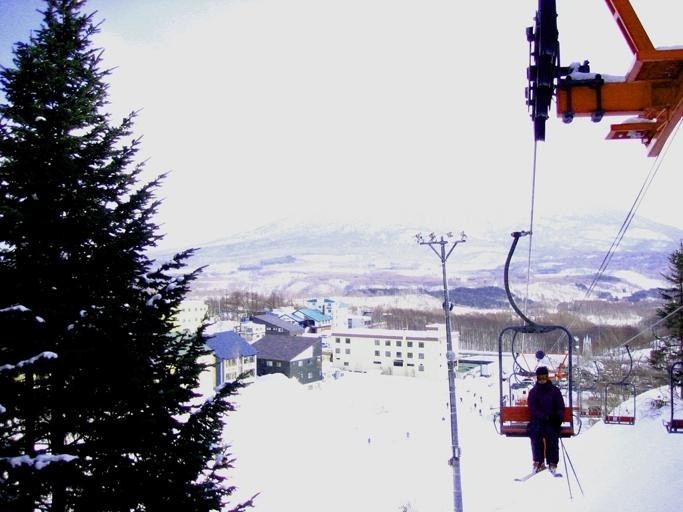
[
  {"xmin": 527, "ymin": 367, "xmax": 564, "ymax": 473},
  {"xmin": 578, "ymin": 60, "xmax": 590, "ymax": 73}
]
